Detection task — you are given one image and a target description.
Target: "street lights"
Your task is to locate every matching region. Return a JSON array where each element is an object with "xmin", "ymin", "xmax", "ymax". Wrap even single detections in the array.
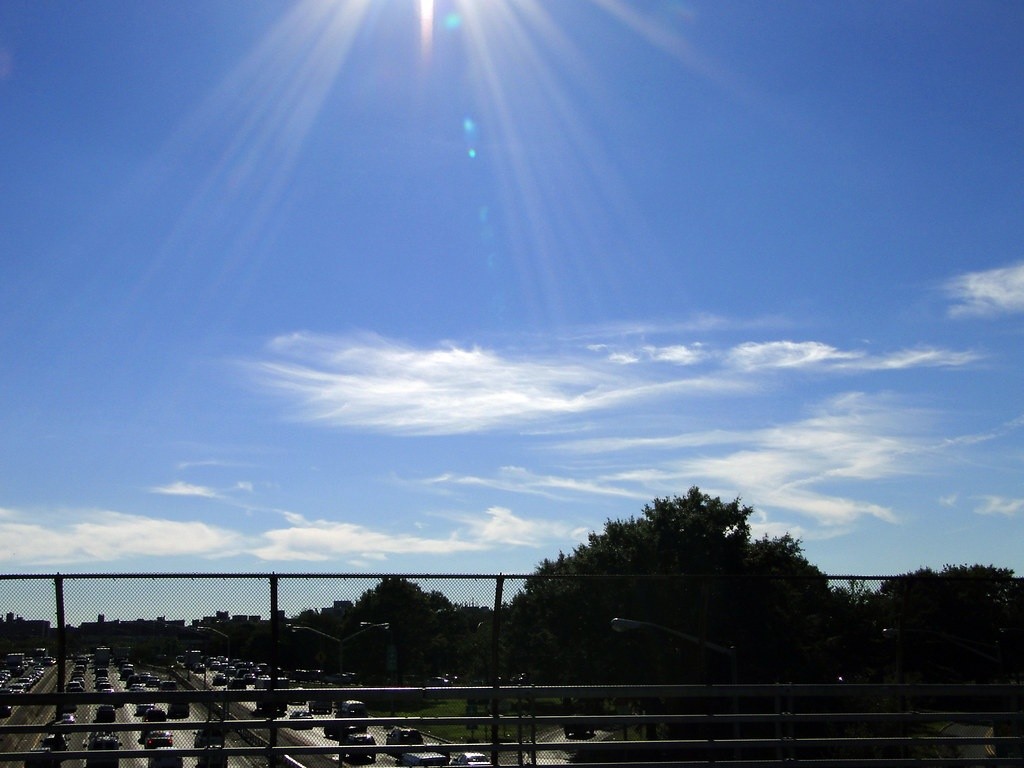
[
  {"xmin": 197, "ymin": 626, "xmax": 230, "ymax": 720},
  {"xmin": 882, "ymin": 624, "xmax": 1008, "ymax": 683},
  {"xmin": 610, "ymin": 616, "xmax": 743, "ymax": 760},
  {"xmin": 289, "ymin": 622, "xmax": 392, "ymax": 768}
]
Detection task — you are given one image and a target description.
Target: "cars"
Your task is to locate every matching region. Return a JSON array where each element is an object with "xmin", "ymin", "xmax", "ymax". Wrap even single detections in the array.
[{"xmin": 0, "ymin": 645, "xmax": 600, "ymax": 768}]
